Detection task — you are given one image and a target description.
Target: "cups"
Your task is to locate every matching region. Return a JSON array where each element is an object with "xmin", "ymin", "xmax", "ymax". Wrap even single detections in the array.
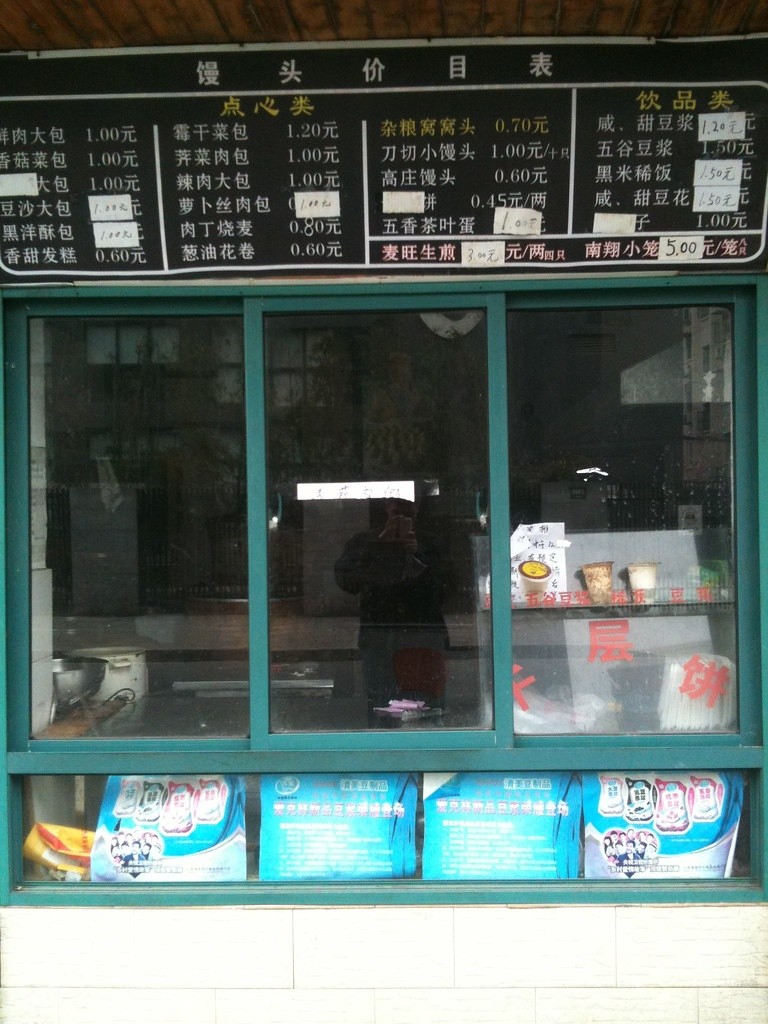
[
  {"xmin": 581, "ymin": 561, "xmax": 613, "ymax": 604},
  {"xmin": 518, "ymin": 560, "xmax": 553, "ymax": 603},
  {"xmin": 627, "ymin": 562, "xmax": 660, "ymax": 604}
]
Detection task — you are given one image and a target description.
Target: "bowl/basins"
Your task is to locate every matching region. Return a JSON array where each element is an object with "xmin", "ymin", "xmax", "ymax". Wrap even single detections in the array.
[{"xmin": 52, "ymin": 657, "xmax": 109, "ymax": 706}]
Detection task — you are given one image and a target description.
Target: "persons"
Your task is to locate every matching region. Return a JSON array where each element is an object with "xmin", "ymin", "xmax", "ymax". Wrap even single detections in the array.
[{"xmin": 336, "ymin": 489, "xmax": 450, "ymax": 728}]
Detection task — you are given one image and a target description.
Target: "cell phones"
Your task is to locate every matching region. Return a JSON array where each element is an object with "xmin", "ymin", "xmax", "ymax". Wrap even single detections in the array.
[{"xmin": 400, "ymin": 518, "xmax": 413, "ymax": 538}]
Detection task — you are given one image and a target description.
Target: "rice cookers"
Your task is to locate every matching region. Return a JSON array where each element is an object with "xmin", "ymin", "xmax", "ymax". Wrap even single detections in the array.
[{"xmin": 64, "ymin": 647, "xmax": 149, "ymax": 704}]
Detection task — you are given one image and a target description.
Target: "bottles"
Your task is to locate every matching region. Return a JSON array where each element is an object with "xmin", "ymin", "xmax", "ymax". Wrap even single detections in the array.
[{"xmin": 55, "ymin": 616, "xmax": 93, "ymax": 648}]
